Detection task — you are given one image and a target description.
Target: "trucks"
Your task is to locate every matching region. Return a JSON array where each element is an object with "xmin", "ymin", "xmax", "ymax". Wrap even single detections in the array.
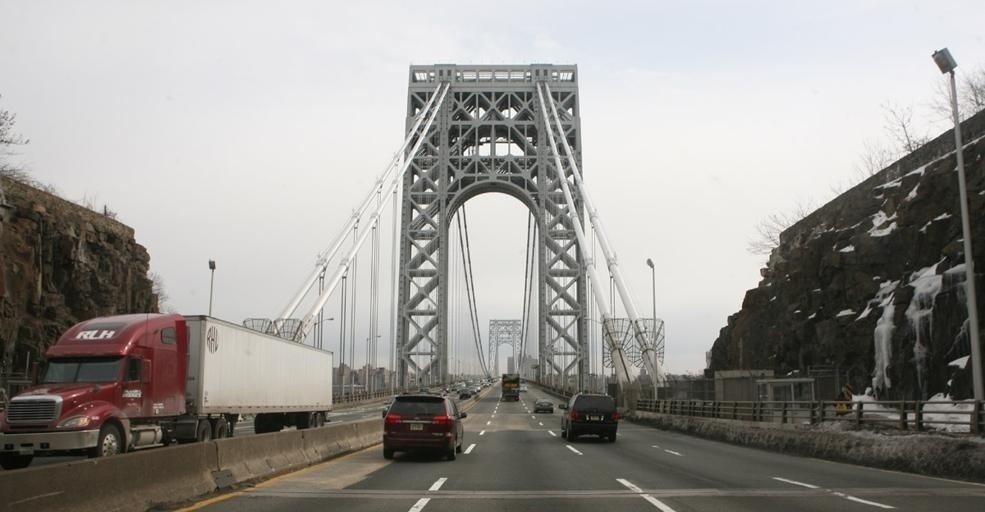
[
  {"xmin": 0, "ymin": 310, "xmax": 336, "ymax": 469},
  {"xmin": 501, "ymin": 373, "xmax": 520, "ymax": 401}
]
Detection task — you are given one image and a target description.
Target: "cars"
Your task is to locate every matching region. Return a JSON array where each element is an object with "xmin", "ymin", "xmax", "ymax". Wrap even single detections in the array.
[
  {"xmin": 380, "ymin": 394, "xmax": 403, "ymax": 418},
  {"xmin": 532, "ymin": 398, "xmax": 554, "ymax": 414},
  {"xmin": 419, "ymin": 375, "xmax": 501, "ymax": 399}
]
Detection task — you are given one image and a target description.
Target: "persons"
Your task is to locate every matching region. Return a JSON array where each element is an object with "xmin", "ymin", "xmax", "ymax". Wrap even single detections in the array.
[{"xmin": 833, "ymin": 389, "xmax": 853, "ymax": 416}]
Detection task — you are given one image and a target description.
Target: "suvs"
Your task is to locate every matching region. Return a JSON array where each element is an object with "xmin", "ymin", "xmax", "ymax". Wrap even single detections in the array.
[
  {"xmin": 557, "ymin": 391, "xmax": 618, "ymax": 443},
  {"xmin": 380, "ymin": 391, "xmax": 468, "ymax": 462}
]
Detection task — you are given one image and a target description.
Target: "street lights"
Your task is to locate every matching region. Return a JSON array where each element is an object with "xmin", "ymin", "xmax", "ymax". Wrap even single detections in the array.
[
  {"xmin": 927, "ymin": 43, "xmax": 985, "ymax": 401},
  {"xmin": 364, "ymin": 336, "xmax": 382, "ymax": 392},
  {"xmin": 313, "ymin": 317, "xmax": 334, "ymax": 346},
  {"xmin": 644, "ymin": 257, "xmax": 657, "ymax": 400},
  {"xmin": 206, "ymin": 259, "xmax": 217, "ymax": 315}
]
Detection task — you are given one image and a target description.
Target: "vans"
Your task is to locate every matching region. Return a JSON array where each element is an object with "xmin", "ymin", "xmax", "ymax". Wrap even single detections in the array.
[{"xmin": 519, "ymin": 378, "xmax": 527, "ymax": 392}]
